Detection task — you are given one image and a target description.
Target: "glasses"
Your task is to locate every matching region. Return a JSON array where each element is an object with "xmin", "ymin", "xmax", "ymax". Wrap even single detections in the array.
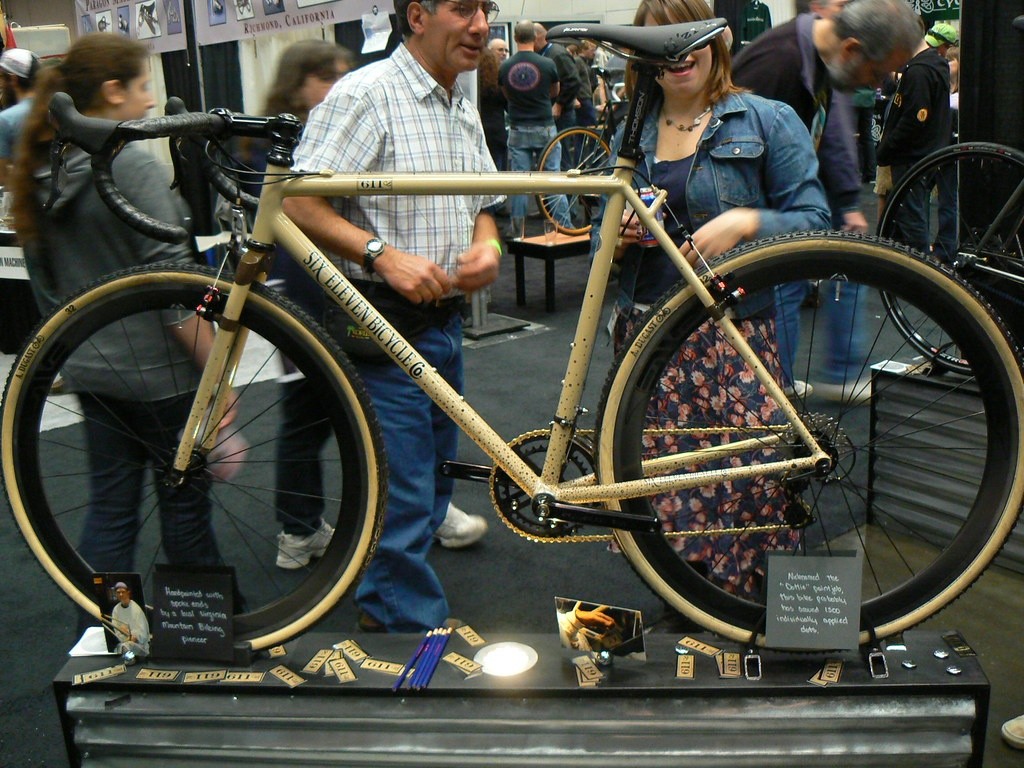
[
  {"xmin": 493, "ymin": 48, "xmax": 508, "ymax": 52},
  {"xmin": 446, "ymin": 0, "xmax": 500, "ymax": 24},
  {"xmin": 928, "ymin": 28, "xmax": 953, "ymax": 46}
]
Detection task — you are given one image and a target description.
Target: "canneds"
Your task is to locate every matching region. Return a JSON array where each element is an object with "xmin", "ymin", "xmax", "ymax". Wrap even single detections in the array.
[{"xmin": 633, "ymin": 186, "xmax": 665, "ymax": 248}]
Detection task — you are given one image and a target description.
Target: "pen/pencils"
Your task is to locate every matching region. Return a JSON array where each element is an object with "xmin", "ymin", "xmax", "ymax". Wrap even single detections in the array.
[{"xmin": 391, "ymin": 626, "xmax": 452, "ymax": 691}]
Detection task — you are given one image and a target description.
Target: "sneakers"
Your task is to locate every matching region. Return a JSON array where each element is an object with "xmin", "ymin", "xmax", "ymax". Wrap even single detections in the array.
[
  {"xmin": 433, "ymin": 501, "xmax": 490, "ymax": 548},
  {"xmin": 277, "ymin": 515, "xmax": 336, "ymax": 570}
]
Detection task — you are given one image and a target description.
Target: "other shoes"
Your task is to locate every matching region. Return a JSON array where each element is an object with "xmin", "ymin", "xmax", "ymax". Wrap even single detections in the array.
[
  {"xmin": 642, "ymin": 604, "xmax": 707, "ymax": 635},
  {"xmin": 354, "ymin": 609, "xmax": 388, "ymax": 636},
  {"xmin": 784, "ymin": 377, "xmax": 873, "ymax": 401}
]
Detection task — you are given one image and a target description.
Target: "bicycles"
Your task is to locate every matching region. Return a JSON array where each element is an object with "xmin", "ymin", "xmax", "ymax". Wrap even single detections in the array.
[
  {"xmin": 534, "ymin": 64, "xmax": 631, "ymax": 236},
  {"xmin": 872, "ymin": 139, "xmax": 1024, "ymax": 382},
  {"xmin": 2, "ymin": 17, "xmax": 1024, "ymax": 666}
]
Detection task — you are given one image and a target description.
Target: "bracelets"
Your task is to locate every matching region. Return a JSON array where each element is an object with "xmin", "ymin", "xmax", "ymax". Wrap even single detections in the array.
[{"xmin": 481, "ymin": 239, "xmax": 503, "ymax": 256}]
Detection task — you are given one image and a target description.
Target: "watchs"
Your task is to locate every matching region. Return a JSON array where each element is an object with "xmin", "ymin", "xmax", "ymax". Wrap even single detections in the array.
[{"xmin": 361, "ymin": 236, "xmax": 388, "ymax": 282}]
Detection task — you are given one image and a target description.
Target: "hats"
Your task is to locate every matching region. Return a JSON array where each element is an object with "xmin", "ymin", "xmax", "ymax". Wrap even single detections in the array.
[
  {"xmin": 923, "ymin": 23, "xmax": 957, "ymax": 47},
  {"xmin": 0, "ymin": 48, "xmax": 43, "ymax": 80}
]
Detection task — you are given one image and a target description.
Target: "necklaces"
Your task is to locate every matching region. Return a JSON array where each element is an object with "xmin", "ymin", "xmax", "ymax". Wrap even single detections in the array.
[{"xmin": 661, "ymin": 96, "xmax": 716, "ymax": 133}]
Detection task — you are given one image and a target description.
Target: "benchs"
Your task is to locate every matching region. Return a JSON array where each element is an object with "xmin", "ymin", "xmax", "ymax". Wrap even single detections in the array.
[{"xmin": 506, "ymin": 226, "xmax": 592, "ymax": 315}]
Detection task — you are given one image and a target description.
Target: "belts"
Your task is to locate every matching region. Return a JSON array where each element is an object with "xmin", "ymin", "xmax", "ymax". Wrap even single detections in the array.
[{"xmin": 349, "ymin": 276, "xmax": 466, "ymax": 308}]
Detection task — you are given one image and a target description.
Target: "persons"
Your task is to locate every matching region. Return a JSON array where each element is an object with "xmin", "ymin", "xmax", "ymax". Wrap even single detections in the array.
[
  {"xmin": 588, "ymin": 0, "xmax": 839, "ymax": 644},
  {"xmin": 557, "ymin": 608, "xmax": 643, "ymax": 651},
  {"xmin": 111, "ymin": 581, "xmax": 151, "ymax": 658},
  {"xmin": 277, "ymin": 0, "xmax": 511, "ymax": 637},
  {"xmin": 1, "ymin": 31, "xmax": 275, "ymax": 641},
  {"xmin": 0, "ymin": 0, "xmax": 964, "ymax": 406},
  {"xmin": 238, "ymin": 37, "xmax": 356, "ymax": 571}
]
